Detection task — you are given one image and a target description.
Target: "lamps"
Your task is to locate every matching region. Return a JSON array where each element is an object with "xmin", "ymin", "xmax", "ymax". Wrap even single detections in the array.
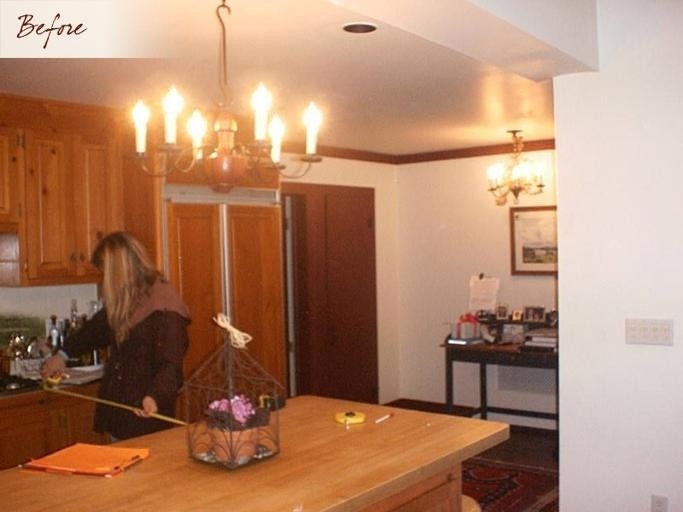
[{"xmin": 131, "ymin": 0, "xmax": 325, "ymax": 195}]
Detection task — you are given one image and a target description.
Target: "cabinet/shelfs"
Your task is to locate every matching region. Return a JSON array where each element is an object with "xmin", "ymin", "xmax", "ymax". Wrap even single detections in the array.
[
  {"xmin": 166, "ymin": 201, "xmax": 288, "ymax": 426},
  {"xmin": 0, "ymin": 398, "xmax": 111, "ymax": 470},
  {"xmin": 0, "ymin": 125, "xmax": 22, "ymax": 226},
  {"xmin": 23, "ymin": 129, "xmax": 120, "ymax": 281},
  {"xmin": 118, "ymin": 132, "xmax": 157, "ymax": 270}
]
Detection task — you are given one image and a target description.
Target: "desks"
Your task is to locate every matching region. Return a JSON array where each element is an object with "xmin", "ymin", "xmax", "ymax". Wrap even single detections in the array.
[
  {"xmin": 439, "ymin": 339, "xmax": 558, "ymax": 430},
  {"xmin": 4, "ymin": 392, "xmax": 510, "ymax": 512}
]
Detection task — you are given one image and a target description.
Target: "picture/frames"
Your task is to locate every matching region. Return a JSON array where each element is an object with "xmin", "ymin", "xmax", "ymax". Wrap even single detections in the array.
[{"xmin": 510, "ymin": 205, "xmax": 557, "ymax": 275}]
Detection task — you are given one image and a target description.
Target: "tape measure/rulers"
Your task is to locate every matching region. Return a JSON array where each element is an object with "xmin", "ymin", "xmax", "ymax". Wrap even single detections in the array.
[{"xmin": 41, "ymin": 372, "xmax": 187, "ymax": 426}]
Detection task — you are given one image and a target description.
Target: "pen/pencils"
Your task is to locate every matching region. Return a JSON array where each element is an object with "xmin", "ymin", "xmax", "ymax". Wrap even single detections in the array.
[{"xmin": 374, "ymin": 411, "xmax": 395, "ymax": 424}]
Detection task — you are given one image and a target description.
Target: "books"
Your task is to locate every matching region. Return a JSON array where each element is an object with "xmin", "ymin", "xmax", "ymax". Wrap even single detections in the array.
[
  {"xmin": 524, "ymin": 327, "xmax": 557, "ymax": 354},
  {"xmin": 19, "ymin": 440, "xmax": 154, "ymax": 479}
]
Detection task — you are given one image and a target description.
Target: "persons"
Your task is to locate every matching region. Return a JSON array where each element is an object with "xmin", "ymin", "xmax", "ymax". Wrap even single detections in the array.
[{"xmin": 39, "ymin": 231, "xmax": 188, "ymax": 437}]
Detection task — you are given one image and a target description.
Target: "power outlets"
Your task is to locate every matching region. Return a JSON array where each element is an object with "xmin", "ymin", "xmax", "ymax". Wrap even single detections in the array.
[{"xmin": 486, "ymin": 129, "xmax": 547, "ymax": 206}]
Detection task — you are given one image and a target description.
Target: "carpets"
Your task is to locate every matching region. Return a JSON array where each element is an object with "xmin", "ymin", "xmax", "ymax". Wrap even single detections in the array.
[{"xmin": 461, "ymin": 453, "xmax": 557, "ymax": 512}]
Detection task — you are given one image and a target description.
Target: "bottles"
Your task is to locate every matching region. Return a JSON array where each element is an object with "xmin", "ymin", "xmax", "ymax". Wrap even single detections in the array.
[{"xmin": 47, "ymin": 299, "xmax": 102, "ymax": 366}]
